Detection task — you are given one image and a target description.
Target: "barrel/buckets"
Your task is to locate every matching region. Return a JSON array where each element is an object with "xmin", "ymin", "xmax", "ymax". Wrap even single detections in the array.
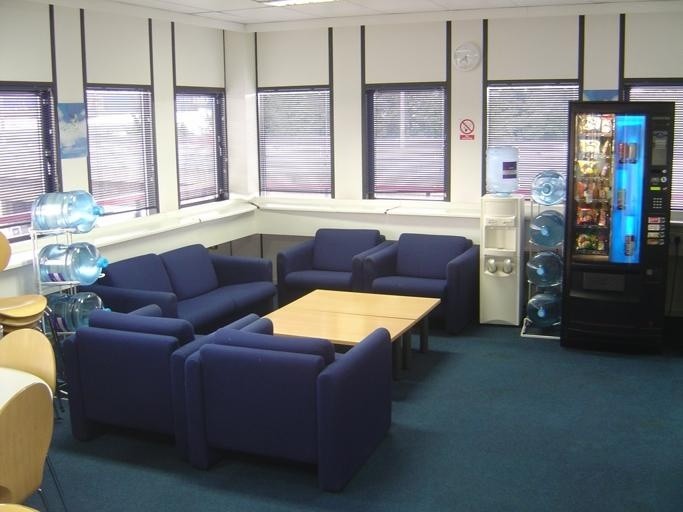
[
  {"xmin": 37, "ymin": 242, "xmax": 108, "ymax": 286},
  {"xmin": 41, "ymin": 294, "xmax": 112, "ymax": 332},
  {"xmin": 31, "ymin": 191, "xmax": 105, "ymax": 233},
  {"xmin": 531, "ymin": 171, "xmax": 568, "ymax": 206},
  {"xmin": 525, "ymin": 251, "xmax": 564, "ymax": 288},
  {"xmin": 44, "ymin": 334, "xmax": 71, "ymax": 383},
  {"xmin": 528, "ymin": 209, "xmax": 566, "ymax": 247},
  {"xmin": 528, "ymin": 290, "xmax": 562, "ymax": 328},
  {"xmin": 485, "ymin": 145, "xmax": 521, "ymax": 196}
]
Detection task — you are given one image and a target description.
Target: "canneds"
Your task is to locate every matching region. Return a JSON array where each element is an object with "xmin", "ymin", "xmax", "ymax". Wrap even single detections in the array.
[
  {"xmin": 625, "ymin": 235, "xmax": 634, "ymax": 256},
  {"xmin": 618, "ymin": 143, "xmax": 628, "ymax": 163},
  {"xmin": 628, "ymin": 143, "xmax": 636, "ymax": 163},
  {"xmin": 617, "ymin": 191, "xmax": 625, "ymax": 210}
]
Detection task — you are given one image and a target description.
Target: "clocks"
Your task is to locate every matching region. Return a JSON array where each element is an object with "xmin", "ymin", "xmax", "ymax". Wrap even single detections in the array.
[{"xmin": 452, "ymin": 42, "xmax": 481, "ymax": 73}]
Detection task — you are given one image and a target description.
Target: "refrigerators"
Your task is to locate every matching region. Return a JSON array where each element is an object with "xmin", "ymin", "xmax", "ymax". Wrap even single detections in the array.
[{"xmin": 558, "ymin": 99, "xmax": 675, "ymax": 356}]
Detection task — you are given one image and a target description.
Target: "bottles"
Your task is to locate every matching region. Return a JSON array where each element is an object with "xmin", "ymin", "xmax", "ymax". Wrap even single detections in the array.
[{"xmin": 573, "ymin": 113, "xmax": 616, "ymax": 262}]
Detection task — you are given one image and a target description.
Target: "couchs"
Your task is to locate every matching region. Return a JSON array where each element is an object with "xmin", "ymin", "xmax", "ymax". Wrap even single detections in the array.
[
  {"xmin": 77, "ymin": 243, "xmax": 276, "ymax": 330},
  {"xmin": 183, "ymin": 317, "xmax": 396, "ymax": 494},
  {"xmin": 60, "ymin": 304, "xmax": 258, "ymax": 464}
]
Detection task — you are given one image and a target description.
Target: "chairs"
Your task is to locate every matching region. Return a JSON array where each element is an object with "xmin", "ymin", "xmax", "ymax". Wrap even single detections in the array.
[
  {"xmin": 0, "ymin": 231, "xmax": 62, "ymax": 365},
  {"xmin": 1, "ymin": 327, "xmax": 71, "ymax": 511},
  {"xmin": 274, "ymin": 227, "xmax": 479, "ymax": 336}
]
